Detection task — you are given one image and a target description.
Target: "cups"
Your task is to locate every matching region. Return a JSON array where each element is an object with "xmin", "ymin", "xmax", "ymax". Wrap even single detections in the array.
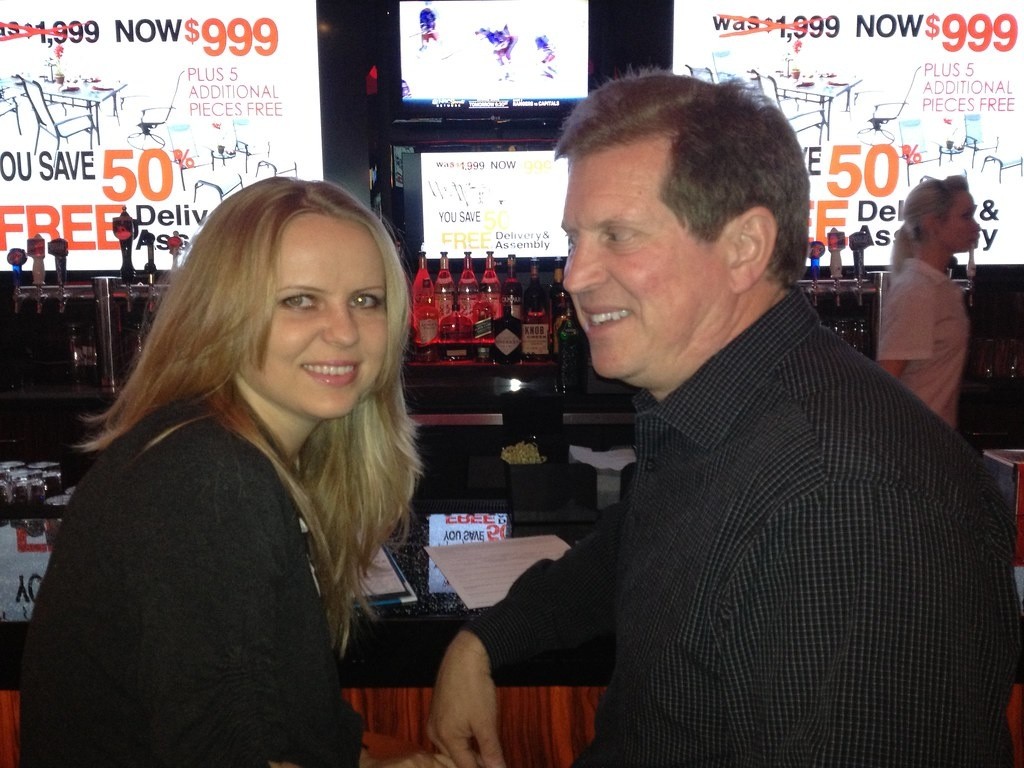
[{"xmin": 0, "ymin": 461, "xmax": 64, "ymax": 503}]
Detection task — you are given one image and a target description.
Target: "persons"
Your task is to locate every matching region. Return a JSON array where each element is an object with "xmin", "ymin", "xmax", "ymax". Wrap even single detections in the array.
[
  {"xmin": 423, "ymin": 68, "xmax": 1024, "ymax": 768},
  {"xmin": 874, "ymin": 174, "xmax": 981, "ymax": 428},
  {"xmin": 18, "ymin": 177, "xmax": 462, "ymax": 768}
]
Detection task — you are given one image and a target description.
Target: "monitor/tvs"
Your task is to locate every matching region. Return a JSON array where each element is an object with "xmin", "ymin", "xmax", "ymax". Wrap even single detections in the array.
[
  {"xmin": 402, "ymin": 150, "xmax": 575, "ymax": 272},
  {"xmin": 387, "ymin": 0, "xmax": 602, "ymax": 129}
]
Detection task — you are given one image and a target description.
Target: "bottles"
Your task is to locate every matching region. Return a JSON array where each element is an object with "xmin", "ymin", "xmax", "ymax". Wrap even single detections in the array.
[{"xmin": 412, "ymin": 250, "xmax": 586, "ymax": 393}]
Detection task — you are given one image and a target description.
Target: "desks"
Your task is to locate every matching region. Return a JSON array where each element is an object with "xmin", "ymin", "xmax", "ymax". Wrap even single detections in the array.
[{"xmin": 0, "ymin": 423, "xmax": 636, "ymax": 768}]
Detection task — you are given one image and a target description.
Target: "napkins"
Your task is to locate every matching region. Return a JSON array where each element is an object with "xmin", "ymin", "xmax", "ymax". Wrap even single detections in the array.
[{"xmin": 568, "ymin": 445, "xmax": 635, "ymax": 471}]
